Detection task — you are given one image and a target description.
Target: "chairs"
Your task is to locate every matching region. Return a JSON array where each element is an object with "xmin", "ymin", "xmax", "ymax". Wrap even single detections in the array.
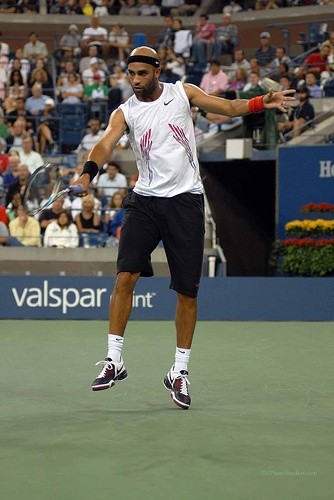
[
  {"xmin": 58, "ymin": 100, "xmax": 87, "ymax": 151},
  {"xmin": 297, "ymin": 23, "xmax": 329, "ymax": 51}
]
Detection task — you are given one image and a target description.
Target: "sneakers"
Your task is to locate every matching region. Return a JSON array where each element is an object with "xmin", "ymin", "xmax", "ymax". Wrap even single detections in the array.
[
  {"xmin": 163, "ymin": 365, "xmax": 192, "ymax": 409},
  {"xmin": 91, "ymin": 356, "xmax": 128, "ymax": 391}
]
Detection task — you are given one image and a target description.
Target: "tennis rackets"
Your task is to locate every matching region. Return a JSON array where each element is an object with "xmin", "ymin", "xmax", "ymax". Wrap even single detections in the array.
[{"xmin": 22, "ymin": 162, "xmax": 88, "ymax": 217}]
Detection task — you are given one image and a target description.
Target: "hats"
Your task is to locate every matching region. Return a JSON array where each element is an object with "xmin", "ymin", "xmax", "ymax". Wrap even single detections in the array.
[
  {"xmin": 93, "ymin": 73, "xmax": 101, "ymax": 80},
  {"xmin": 297, "ymin": 84, "xmax": 309, "ymax": 92},
  {"xmin": 89, "ymin": 57, "xmax": 103, "ymax": 66},
  {"xmin": 260, "ymin": 32, "xmax": 270, "ymax": 39}
]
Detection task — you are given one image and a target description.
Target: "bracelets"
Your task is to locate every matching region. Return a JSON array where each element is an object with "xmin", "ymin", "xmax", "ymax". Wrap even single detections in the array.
[
  {"xmin": 80, "ymin": 161, "xmax": 98, "ymax": 183},
  {"xmin": 248, "ymin": 95, "xmax": 267, "ymax": 113}
]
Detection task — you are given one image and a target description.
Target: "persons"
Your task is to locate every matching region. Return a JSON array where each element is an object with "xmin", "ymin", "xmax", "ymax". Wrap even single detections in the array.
[
  {"xmin": 0, "ymin": 0, "xmax": 334, "ymax": 249},
  {"xmin": 68, "ymin": 46, "xmax": 298, "ymax": 411}
]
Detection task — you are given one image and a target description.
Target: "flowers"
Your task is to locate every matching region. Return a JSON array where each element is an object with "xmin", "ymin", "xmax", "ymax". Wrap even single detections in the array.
[{"xmin": 270, "ymin": 202, "xmax": 334, "ymax": 278}]
